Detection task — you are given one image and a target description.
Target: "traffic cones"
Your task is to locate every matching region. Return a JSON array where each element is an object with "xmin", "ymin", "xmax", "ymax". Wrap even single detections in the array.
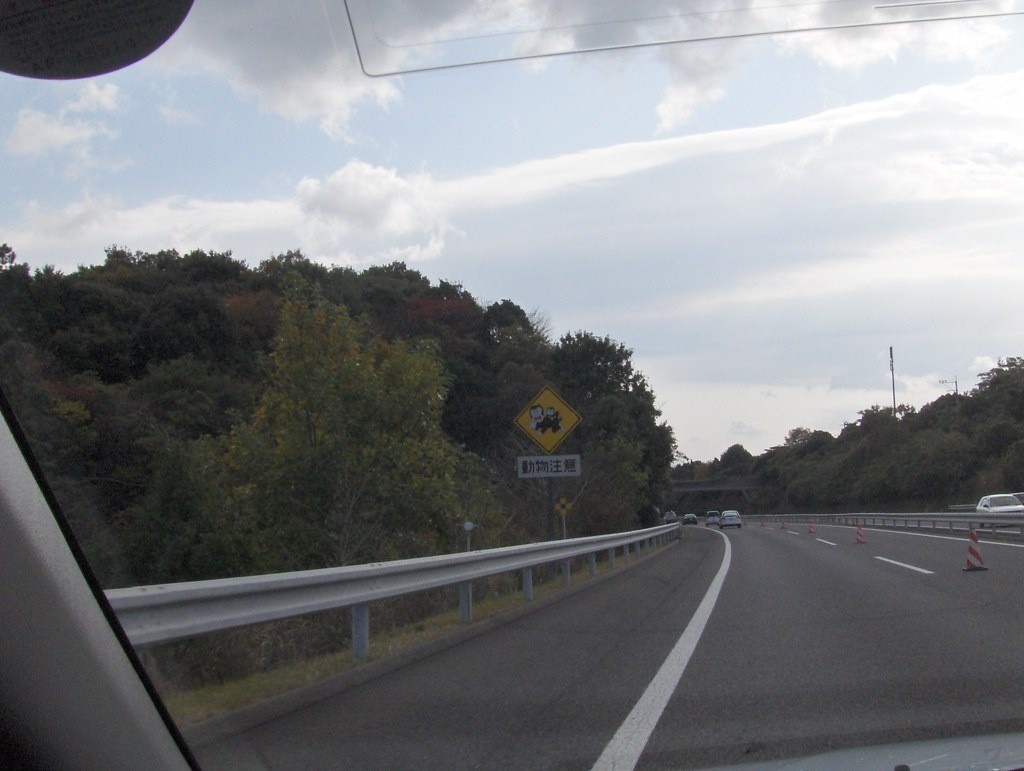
[
  {"xmin": 807, "ymin": 519, "xmax": 816, "ymax": 534},
  {"xmin": 781, "ymin": 517, "xmax": 788, "ymax": 530},
  {"xmin": 852, "ymin": 524, "xmax": 869, "ymax": 546},
  {"xmin": 961, "ymin": 526, "xmax": 989, "ymax": 569}
]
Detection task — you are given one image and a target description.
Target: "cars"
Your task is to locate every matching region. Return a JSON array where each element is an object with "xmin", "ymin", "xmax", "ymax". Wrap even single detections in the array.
[
  {"xmin": 704, "ymin": 510, "xmax": 720, "ymax": 527},
  {"xmin": 663, "ymin": 511, "xmax": 678, "ymax": 523},
  {"xmin": 976, "ymin": 493, "xmax": 1024, "ymax": 528},
  {"xmin": 719, "ymin": 510, "xmax": 742, "ymax": 529},
  {"xmin": 682, "ymin": 514, "xmax": 698, "ymax": 527}
]
